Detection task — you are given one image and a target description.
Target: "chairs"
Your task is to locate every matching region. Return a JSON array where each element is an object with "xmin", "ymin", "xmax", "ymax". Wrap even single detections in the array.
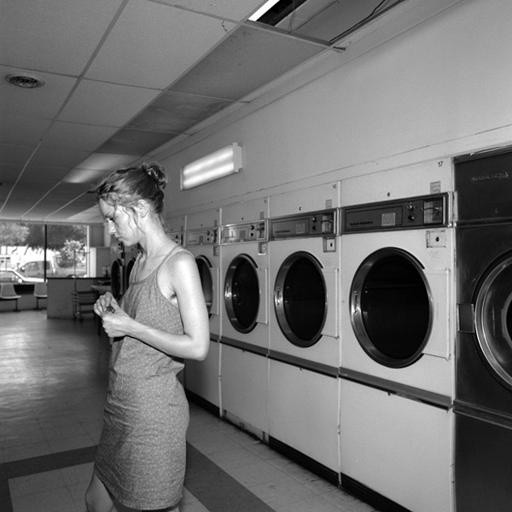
[
  {"xmin": 0, "ymin": 282, "xmax": 22, "ymax": 312},
  {"xmin": 33, "ymin": 282, "xmax": 48, "ymax": 310}
]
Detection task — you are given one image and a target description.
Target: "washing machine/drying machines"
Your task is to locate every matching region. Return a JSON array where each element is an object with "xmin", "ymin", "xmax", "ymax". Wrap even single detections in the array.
[
  {"xmin": 101, "ymin": 214, "xmax": 184, "ymax": 398},
  {"xmin": 185, "ymin": 146, "xmax": 511, "ymax": 512}
]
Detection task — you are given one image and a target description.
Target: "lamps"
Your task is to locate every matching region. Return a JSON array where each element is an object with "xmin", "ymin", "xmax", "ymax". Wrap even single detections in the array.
[{"xmin": 180, "ymin": 142, "xmax": 242, "ymax": 191}]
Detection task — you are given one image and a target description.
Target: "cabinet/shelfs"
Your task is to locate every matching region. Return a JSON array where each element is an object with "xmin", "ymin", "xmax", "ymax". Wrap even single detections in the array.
[
  {"xmin": 89, "ymin": 246, "xmax": 111, "ymax": 278},
  {"xmin": 47, "ymin": 277, "xmax": 112, "ymax": 320}
]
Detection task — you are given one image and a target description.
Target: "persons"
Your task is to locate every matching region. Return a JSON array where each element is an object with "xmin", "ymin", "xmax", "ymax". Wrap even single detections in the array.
[{"xmin": 85, "ymin": 161, "xmax": 212, "ymax": 511}]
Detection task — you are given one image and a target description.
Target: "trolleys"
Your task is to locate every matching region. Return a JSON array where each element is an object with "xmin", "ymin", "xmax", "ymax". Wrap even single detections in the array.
[{"xmin": 70, "ymin": 277, "xmax": 100, "ymax": 322}]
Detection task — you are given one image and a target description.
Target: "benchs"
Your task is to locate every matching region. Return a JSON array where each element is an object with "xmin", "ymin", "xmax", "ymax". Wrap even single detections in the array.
[{"xmin": 71, "ymin": 290, "xmax": 99, "ymax": 321}]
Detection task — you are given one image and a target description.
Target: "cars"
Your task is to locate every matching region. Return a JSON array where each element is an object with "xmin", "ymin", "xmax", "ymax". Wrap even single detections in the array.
[{"xmin": 0, "ymin": 254, "xmax": 87, "ymax": 298}]
